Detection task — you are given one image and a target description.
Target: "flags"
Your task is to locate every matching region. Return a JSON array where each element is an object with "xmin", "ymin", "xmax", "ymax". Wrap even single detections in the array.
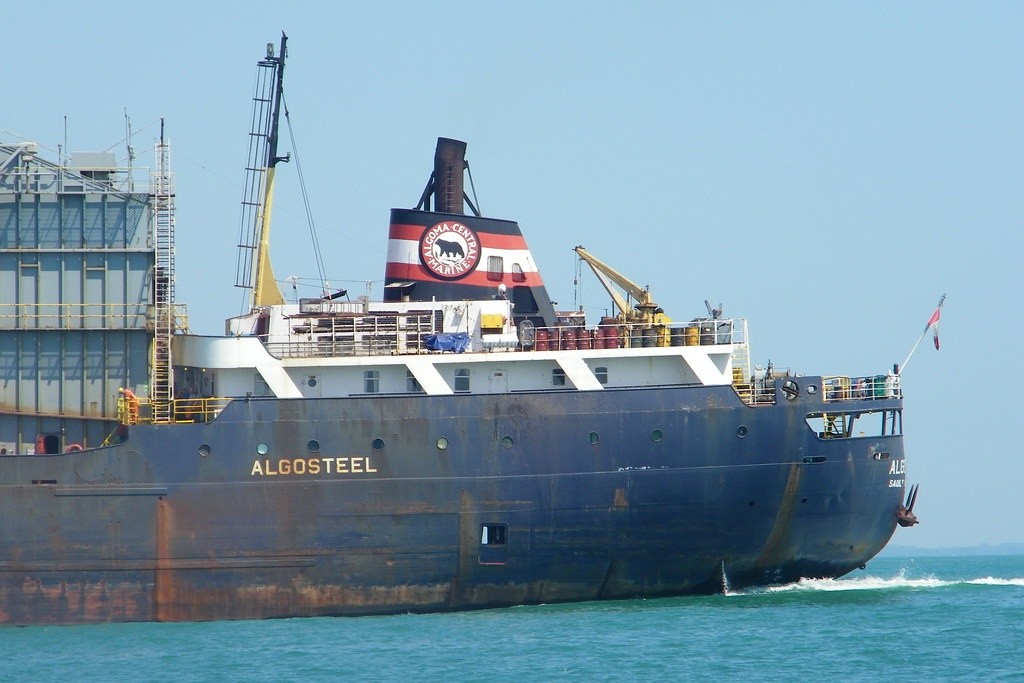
[{"xmin": 927, "ymin": 306, "xmax": 939, "ymax": 350}]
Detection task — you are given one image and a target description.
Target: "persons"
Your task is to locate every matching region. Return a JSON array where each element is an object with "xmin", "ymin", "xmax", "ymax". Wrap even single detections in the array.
[
  {"xmin": 118, "ymin": 387, "xmax": 137, "ymax": 425},
  {"xmin": 116, "ymin": 420, "xmax": 126, "ymax": 443},
  {"xmin": 174, "ymin": 386, "xmax": 204, "ymax": 422},
  {"xmin": 257, "ymin": 312, "xmax": 266, "ymax": 333}
]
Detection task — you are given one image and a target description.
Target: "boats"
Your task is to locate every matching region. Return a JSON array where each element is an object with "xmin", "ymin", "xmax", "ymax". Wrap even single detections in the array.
[{"xmin": 1, "ymin": 28, "xmax": 905, "ymax": 626}]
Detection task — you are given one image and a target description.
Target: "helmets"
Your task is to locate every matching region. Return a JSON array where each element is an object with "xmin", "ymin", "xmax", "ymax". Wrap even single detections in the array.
[{"xmin": 119, "ymin": 387, "xmax": 123, "ymax": 394}]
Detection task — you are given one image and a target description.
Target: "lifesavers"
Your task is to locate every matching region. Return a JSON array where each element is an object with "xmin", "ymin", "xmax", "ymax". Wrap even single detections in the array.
[{"xmin": 67, "ymin": 444, "xmax": 82, "ymax": 453}]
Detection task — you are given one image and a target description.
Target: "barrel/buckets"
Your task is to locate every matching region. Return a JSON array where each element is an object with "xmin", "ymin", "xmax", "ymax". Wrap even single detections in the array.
[
  {"xmin": 629, "ymin": 329, "xmax": 642, "ymax": 348},
  {"xmin": 656, "ymin": 328, "xmax": 670, "ymax": 347},
  {"xmin": 642, "ymin": 329, "xmax": 656, "ymax": 347},
  {"xmin": 563, "ymin": 331, "xmax": 577, "ymax": 350},
  {"xmin": 686, "ymin": 327, "xmax": 700, "ymax": 346},
  {"xmin": 670, "ymin": 328, "xmax": 685, "ymax": 346},
  {"xmin": 701, "ymin": 327, "xmax": 715, "ymax": 345},
  {"xmin": 577, "ymin": 330, "xmax": 591, "ymax": 350},
  {"xmin": 536, "ymin": 331, "xmax": 549, "ymax": 351},
  {"xmin": 606, "ymin": 329, "xmax": 619, "ymax": 348},
  {"xmin": 591, "ymin": 329, "xmax": 605, "ymax": 349},
  {"xmin": 548, "ymin": 331, "xmax": 559, "ymax": 350}
]
